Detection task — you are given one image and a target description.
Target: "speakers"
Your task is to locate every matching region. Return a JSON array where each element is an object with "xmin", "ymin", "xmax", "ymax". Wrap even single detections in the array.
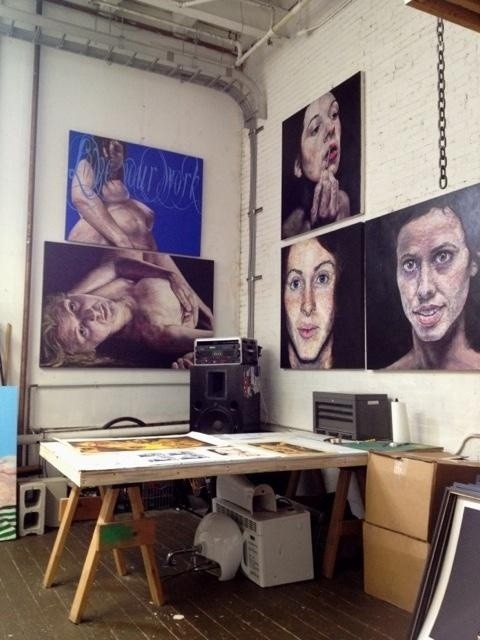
[{"xmin": 189, "ymin": 365, "xmax": 260, "ymax": 435}]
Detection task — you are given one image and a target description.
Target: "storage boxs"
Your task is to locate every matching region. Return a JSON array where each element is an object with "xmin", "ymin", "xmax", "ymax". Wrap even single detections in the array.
[{"xmin": 362, "ymin": 450, "xmax": 480, "ymax": 615}]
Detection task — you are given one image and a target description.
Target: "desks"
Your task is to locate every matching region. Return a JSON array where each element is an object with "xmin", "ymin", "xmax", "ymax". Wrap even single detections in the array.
[{"xmin": 39, "ymin": 430, "xmax": 444, "ymax": 626}]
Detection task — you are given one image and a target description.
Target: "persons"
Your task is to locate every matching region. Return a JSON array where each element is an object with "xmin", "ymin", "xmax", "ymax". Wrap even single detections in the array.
[
  {"xmin": 67, "ymin": 139, "xmax": 157, "ymax": 250},
  {"xmin": 283, "ymin": 238, "xmax": 337, "ymax": 369},
  {"xmin": 375, "ymin": 195, "xmax": 480, "ymax": 369},
  {"xmin": 41, "ymin": 250, "xmax": 214, "ymax": 369},
  {"xmin": 282, "ymin": 92, "xmax": 350, "ymax": 238}
]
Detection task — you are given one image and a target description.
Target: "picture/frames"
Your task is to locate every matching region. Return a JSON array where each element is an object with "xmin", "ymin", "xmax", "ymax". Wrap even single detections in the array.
[{"xmin": 405, "ymin": 482, "xmax": 480, "ymax": 640}]
